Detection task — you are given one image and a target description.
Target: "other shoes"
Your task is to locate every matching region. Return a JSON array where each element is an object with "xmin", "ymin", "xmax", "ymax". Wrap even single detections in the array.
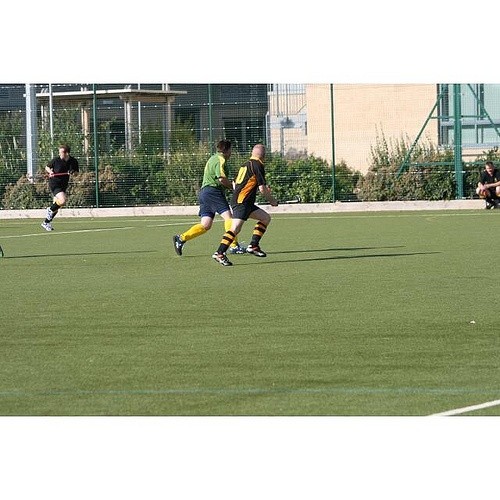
[{"xmin": 486, "ymin": 201, "xmax": 496, "ymax": 209}]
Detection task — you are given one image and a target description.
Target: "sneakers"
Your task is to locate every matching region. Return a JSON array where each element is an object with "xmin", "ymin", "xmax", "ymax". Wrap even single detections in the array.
[
  {"xmin": 41, "ymin": 221, "xmax": 55, "ymax": 231},
  {"xmin": 211, "ymin": 251, "xmax": 232, "ymax": 266},
  {"xmin": 47, "ymin": 207, "xmax": 53, "ymax": 222},
  {"xmin": 229, "ymin": 246, "xmax": 249, "ymax": 255},
  {"xmin": 246, "ymin": 244, "xmax": 266, "ymax": 257},
  {"xmin": 173, "ymin": 234, "xmax": 186, "ymax": 256}
]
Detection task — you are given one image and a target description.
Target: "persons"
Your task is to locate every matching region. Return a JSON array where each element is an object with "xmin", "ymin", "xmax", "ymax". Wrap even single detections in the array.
[
  {"xmin": 475, "ymin": 161, "xmax": 500, "ymax": 209},
  {"xmin": 212, "ymin": 144, "xmax": 278, "ymax": 266},
  {"xmin": 40, "ymin": 144, "xmax": 79, "ymax": 232},
  {"xmin": 173, "ymin": 140, "xmax": 247, "ymax": 255}
]
